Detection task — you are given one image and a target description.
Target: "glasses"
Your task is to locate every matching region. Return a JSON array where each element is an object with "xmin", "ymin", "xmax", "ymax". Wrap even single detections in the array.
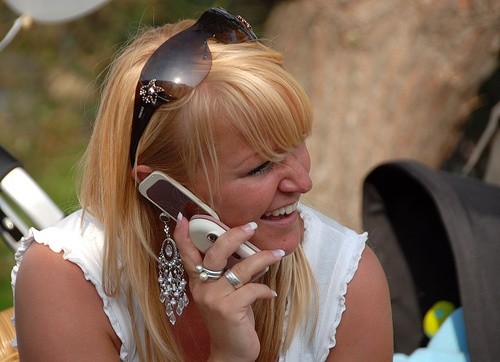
[{"xmin": 130, "ymin": 6, "xmax": 258, "ymax": 169}]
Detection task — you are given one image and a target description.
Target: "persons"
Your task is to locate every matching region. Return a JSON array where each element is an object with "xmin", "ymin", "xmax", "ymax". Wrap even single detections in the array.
[{"xmin": 11, "ymin": 7, "xmax": 395, "ymax": 362}]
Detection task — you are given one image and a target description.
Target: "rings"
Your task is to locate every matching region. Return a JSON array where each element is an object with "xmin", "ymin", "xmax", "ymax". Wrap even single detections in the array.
[
  {"xmin": 225, "ymin": 269, "xmax": 242, "ymax": 289},
  {"xmin": 195, "ymin": 263, "xmax": 220, "ymax": 281}
]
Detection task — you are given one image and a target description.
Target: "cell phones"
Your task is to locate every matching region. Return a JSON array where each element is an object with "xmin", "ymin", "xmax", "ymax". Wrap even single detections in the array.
[{"xmin": 137, "ymin": 169, "xmax": 269, "ymax": 284}]
